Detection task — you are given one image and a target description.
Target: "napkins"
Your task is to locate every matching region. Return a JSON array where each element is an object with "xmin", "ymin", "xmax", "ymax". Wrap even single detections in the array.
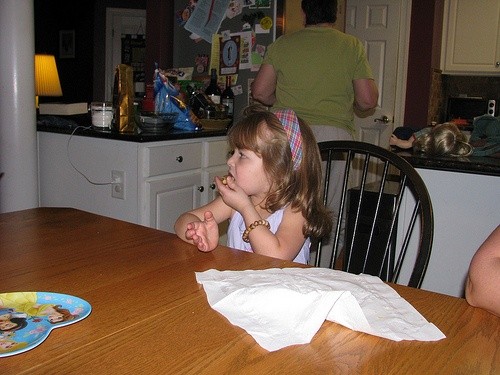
[{"xmin": 192, "ymin": 268, "xmax": 447, "ymax": 354}]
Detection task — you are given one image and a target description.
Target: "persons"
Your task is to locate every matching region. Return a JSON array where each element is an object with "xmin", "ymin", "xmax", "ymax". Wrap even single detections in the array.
[
  {"xmin": 173, "ymin": 108, "xmax": 332, "ymax": 270},
  {"xmin": 252, "ymin": 0, "xmax": 380, "ymax": 266},
  {"xmin": 465, "ymin": 223, "xmax": 500, "ymax": 315}
]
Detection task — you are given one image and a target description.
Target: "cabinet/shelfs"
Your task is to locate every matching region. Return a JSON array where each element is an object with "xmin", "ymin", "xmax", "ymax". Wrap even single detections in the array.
[
  {"xmin": 141, "ymin": 139, "xmax": 233, "ymax": 248},
  {"xmin": 439, "ymin": 0, "xmax": 500, "ymax": 76},
  {"xmin": 342, "ymin": 179, "xmax": 401, "ymax": 282}
]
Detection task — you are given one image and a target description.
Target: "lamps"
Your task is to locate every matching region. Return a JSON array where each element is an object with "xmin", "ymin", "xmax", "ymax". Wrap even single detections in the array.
[{"xmin": 34, "ymin": 54, "xmax": 63, "ymax": 121}]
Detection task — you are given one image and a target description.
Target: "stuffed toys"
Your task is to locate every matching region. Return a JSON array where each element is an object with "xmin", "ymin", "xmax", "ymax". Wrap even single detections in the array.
[{"xmin": 387, "ymin": 123, "xmax": 477, "ymax": 162}]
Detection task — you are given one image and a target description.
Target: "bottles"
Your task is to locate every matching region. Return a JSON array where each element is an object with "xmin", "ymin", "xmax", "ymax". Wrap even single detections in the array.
[
  {"xmin": 204, "ymin": 68, "xmax": 222, "ymax": 105},
  {"xmin": 187, "ymin": 85, "xmax": 213, "ymax": 116},
  {"xmin": 221, "ymin": 76, "xmax": 233, "ymax": 127}
]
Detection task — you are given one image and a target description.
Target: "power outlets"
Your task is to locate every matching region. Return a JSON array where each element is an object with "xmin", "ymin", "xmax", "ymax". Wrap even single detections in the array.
[{"xmin": 110, "ymin": 168, "xmax": 126, "ymax": 200}]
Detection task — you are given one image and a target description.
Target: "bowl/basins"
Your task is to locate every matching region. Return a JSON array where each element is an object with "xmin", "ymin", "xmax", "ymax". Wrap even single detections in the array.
[{"xmin": 136, "ymin": 108, "xmax": 177, "ymax": 136}]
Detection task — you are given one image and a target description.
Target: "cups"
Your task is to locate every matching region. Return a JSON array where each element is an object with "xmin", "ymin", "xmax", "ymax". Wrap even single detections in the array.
[{"xmin": 91, "ymin": 102, "xmax": 114, "ymax": 133}]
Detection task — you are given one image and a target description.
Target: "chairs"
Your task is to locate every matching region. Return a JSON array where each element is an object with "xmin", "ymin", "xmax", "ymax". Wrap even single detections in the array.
[{"xmin": 306, "ymin": 139, "xmax": 436, "ymax": 290}]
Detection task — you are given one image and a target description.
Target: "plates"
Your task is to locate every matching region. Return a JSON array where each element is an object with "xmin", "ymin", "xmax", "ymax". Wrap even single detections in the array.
[{"xmin": 0, "ymin": 291, "xmax": 93, "ymax": 357}]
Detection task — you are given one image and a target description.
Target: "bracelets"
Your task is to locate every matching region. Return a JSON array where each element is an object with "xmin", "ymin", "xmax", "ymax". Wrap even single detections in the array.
[{"xmin": 242, "ymin": 218, "xmax": 271, "ymax": 242}]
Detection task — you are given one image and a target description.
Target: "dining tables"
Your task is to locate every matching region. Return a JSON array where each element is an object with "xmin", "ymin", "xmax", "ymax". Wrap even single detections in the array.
[{"xmin": 1, "ymin": 206, "xmax": 500, "ymax": 375}]
